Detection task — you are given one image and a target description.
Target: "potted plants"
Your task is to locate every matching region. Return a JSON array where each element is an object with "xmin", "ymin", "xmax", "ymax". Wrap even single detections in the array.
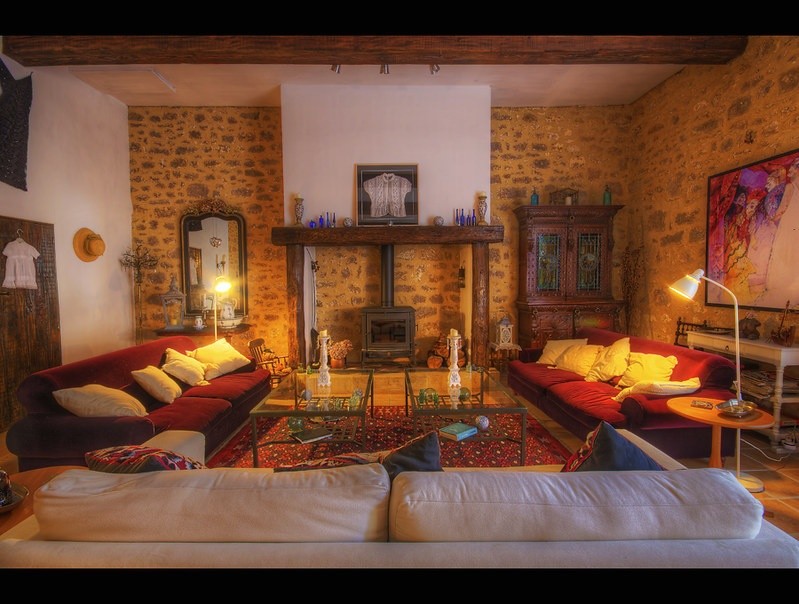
[{"xmin": 329, "ymin": 340, "xmax": 354, "ymax": 369}]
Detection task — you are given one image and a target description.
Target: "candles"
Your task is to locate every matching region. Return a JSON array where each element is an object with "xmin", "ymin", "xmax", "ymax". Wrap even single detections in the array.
[
  {"xmin": 450, "ymin": 327, "xmax": 459, "ymax": 337},
  {"xmin": 320, "ymin": 329, "xmax": 327, "ymax": 338}
]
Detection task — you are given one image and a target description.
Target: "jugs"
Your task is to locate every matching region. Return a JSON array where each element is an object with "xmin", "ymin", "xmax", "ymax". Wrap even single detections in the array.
[{"xmin": 218, "ymin": 298, "xmax": 237, "ymax": 320}]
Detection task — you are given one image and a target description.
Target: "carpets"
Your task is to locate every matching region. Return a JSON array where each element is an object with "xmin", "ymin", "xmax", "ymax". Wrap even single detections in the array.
[{"xmin": 206, "ymin": 403, "xmax": 576, "ymax": 470}]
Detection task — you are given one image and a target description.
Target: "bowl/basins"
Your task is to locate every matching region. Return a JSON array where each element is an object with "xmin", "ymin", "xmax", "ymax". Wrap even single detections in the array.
[
  {"xmin": 716, "ymin": 401, "xmax": 758, "ymax": 418},
  {"xmin": 214, "ymin": 316, "xmax": 244, "ymax": 329}
]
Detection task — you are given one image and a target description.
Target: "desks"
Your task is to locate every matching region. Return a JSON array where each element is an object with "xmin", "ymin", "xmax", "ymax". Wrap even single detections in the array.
[
  {"xmin": 249, "ymin": 367, "xmax": 528, "ymax": 468},
  {"xmin": 667, "ymin": 397, "xmax": 776, "ymax": 468},
  {"xmin": 151, "ymin": 322, "xmax": 252, "ymax": 344}
]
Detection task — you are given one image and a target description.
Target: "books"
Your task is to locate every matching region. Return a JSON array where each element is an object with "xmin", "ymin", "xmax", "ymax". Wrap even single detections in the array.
[
  {"xmin": 740, "ymin": 371, "xmax": 799, "ymax": 395},
  {"xmin": 439, "ymin": 422, "xmax": 477, "ymax": 441}
]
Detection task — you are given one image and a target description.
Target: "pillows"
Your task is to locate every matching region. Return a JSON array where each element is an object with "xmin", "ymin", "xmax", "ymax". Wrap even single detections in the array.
[
  {"xmin": 536, "ymin": 339, "xmax": 699, "ymax": 403},
  {"xmin": 52, "ymin": 337, "xmax": 250, "ymax": 416},
  {"xmin": 83, "ymin": 420, "xmax": 669, "ymax": 486}
]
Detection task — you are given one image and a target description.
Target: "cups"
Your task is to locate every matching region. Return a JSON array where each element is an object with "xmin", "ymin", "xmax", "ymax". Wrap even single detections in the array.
[{"xmin": 195, "ymin": 316, "xmax": 203, "ymax": 330}]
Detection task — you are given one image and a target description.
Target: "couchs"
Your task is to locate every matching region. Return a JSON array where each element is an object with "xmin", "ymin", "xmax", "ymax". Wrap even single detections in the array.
[
  {"xmin": 0, "ymin": 426, "xmax": 799, "ymax": 569},
  {"xmin": 4, "ymin": 336, "xmax": 272, "ymax": 475},
  {"xmin": 508, "ymin": 326, "xmax": 744, "ymax": 458}
]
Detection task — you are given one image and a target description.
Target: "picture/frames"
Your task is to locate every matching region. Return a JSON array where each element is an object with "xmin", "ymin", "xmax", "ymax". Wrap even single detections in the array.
[
  {"xmin": 355, "ymin": 164, "xmax": 419, "ymax": 226},
  {"xmin": 703, "ymin": 147, "xmax": 799, "ymax": 314}
]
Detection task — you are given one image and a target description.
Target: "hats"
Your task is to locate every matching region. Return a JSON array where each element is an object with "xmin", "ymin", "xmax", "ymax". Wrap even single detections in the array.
[{"xmin": 74, "ymin": 228, "xmax": 106, "ymax": 262}]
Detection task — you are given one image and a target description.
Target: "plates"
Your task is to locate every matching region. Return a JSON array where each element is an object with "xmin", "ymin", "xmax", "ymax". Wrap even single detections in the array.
[
  {"xmin": 707, "ymin": 330, "xmax": 730, "ymax": 333},
  {"xmin": 0, "ymin": 481, "xmax": 29, "ymax": 513}
]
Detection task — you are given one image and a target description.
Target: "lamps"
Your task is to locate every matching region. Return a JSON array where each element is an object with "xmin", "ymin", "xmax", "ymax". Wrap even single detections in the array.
[
  {"xmin": 160, "ymin": 271, "xmax": 231, "ymax": 341},
  {"xmin": 669, "ymin": 269, "xmax": 764, "ymax": 493}
]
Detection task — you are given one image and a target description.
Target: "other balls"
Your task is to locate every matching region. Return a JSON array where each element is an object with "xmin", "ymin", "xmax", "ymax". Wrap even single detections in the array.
[
  {"xmin": 301, "ymin": 389, "xmax": 312, "ymax": 400},
  {"xmin": 458, "ymin": 388, "xmax": 471, "ymax": 401},
  {"xmin": 288, "ymin": 416, "xmax": 305, "ymax": 432},
  {"xmin": 423, "ymin": 388, "xmax": 437, "ymax": 403},
  {"xmin": 475, "ymin": 416, "xmax": 489, "ymax": 429}
]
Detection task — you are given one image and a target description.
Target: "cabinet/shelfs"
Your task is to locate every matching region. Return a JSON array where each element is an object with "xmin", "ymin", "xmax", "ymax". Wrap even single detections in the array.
[
  {"xmin": 513, "ymin": 202, "xmax": 631, "ymax": 361},
  {"xmin": 686, "ymin": 330, "xmax": 799, "ymax": 446}
]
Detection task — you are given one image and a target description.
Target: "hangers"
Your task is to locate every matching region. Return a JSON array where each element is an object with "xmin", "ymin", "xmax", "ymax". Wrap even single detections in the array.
[{"xmin": 17, "ymin": 229, "xmax": 25, "ymax": 243}]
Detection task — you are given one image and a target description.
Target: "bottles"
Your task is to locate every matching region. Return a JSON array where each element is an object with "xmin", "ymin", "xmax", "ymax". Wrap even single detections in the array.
[
  {"xmin": 455, "ymin": 208, "xmax": 476, "ymax": 226},
  {"xmin": 309, "ymin": 211, "xmax": 335, "ymax": 228},
  {"xmin": 603, "ymin": 185, "xmax": 611, "ymax": 204},
  {"xmin": 531, "ymin": 186, "xmax": 538, "ymax": 206}
]
